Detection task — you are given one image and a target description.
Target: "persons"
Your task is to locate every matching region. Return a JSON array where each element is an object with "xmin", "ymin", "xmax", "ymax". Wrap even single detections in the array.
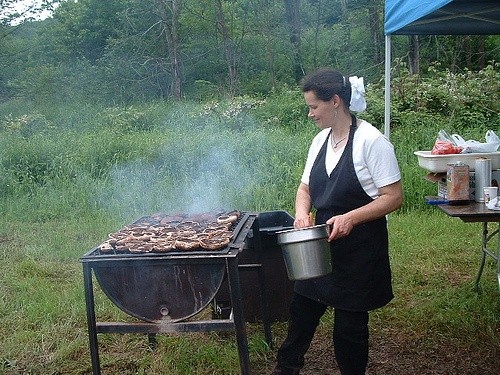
[{"xmin": 270, "ymin": 71, "xmax": 403, "ymax": 375}]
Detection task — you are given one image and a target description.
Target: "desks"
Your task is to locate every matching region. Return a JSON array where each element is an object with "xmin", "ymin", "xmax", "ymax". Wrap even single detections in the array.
[{"xmin": 424, "ymin": 195, "xmax": 500, "ymax": 293}]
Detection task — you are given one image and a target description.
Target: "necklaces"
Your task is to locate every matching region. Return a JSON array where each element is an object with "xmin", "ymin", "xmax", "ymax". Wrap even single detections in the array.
[{"xmin": 333, "ymin": 134, "xmax": 348, "ymax": 148}]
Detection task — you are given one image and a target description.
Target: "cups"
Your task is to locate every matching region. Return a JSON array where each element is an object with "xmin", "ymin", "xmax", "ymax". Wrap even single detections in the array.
[{"xmin": 483, "ymin": 186, "xmax": 498, "ymax": 204}]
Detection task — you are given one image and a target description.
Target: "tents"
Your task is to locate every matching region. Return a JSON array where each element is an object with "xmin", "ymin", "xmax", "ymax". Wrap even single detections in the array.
[{"xmin": 384, "ymin": 0, "xmax": 500, "ymax": 231}]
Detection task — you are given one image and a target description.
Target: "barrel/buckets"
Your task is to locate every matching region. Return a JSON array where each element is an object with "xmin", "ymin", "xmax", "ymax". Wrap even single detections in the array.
[{"xmin": 275, "ymin": 224, "xmax": 333, "ymax": 280}]
